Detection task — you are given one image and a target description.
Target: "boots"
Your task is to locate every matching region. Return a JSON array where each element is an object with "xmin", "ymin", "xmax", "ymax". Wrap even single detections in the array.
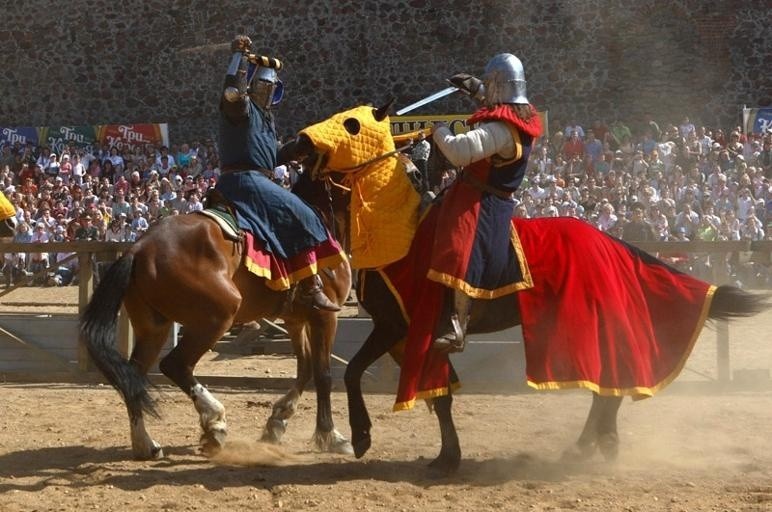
[{"xmin": 431, "ymin": 290, "xmax": 471, "ymax": 353}]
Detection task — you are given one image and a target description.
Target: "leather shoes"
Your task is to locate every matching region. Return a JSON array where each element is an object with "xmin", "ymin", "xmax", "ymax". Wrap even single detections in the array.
[{"xmin": 293, "ymin": 283, "xmax": 341, "ymax": 312}]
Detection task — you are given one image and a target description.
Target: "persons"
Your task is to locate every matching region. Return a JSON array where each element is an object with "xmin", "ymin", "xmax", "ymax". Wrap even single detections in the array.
[
  {"xmin": 1, "ymin": 134, "xmax": 304, "ymax": 285},
  {"xmin": 431, "ymin": 54, "xmax": 544, "ymax": 351},
  {"xmin": 217, "ymin": 34, "xmax": 341, "ymax": 312},
  {"xmin": 411, "ymin": 117, "xmax": 772, "ymax": 287}
]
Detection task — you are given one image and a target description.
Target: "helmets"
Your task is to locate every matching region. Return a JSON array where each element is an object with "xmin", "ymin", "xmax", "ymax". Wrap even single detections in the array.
[
  {"xmin": 475, "ymin": 53, "xmax": 531, "ymax": 106},
  {"xmin": 245, "ymin": 63, "xmax": 285, "ymax": 109}
]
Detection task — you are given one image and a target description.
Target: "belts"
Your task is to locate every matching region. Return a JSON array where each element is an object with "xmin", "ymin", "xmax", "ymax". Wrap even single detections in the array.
[{"xmin": 219, "ymin": 163, "xmax": 274, "ymax": 180}]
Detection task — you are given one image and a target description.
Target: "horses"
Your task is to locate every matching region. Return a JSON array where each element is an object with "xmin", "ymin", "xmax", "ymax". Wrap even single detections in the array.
[
  {"xmin": 0, "ymin": 190, "xmax": 16, "ymax": 246},
  {"xmin": 74, "ymin": 165, "xmax": 350, "ymax": 464},
  {"xmin": 297, "ymin": 96, "xmax": 771, "ymax": 481}
]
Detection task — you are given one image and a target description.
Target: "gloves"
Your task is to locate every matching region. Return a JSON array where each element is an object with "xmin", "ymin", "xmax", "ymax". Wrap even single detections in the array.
[
  {"xmin": 448, "ymin": 72, "xmax": 483, "ymax": 94},
  {"xmin": 231, "ymin": 34, "xmax": 253, "ymax": 55}
]
